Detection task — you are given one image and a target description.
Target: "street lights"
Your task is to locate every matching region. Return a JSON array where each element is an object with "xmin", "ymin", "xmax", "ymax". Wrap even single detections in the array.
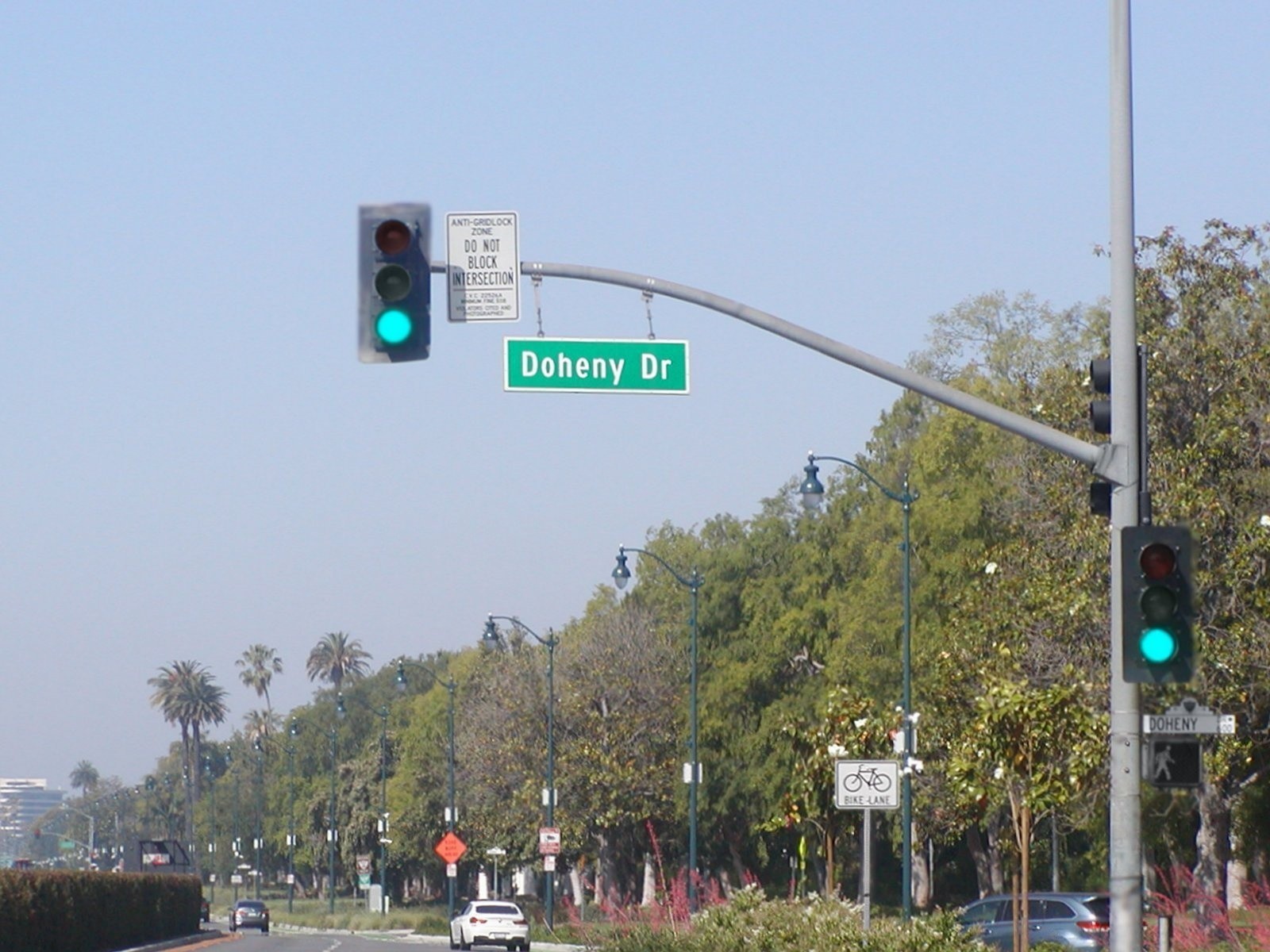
[
  {"xmin": 393, "ymin": 659, "xmax": 457, "ymax": 923},
  {"xmin": 204, "ymin": 757, "xmax": 241, "ymax": 904},
  {"xmin": 251, "ymin": 731, "xmax": 295, "ymax": 914},
  {"xmin": 483, "ymin": 614, "xmax": 558, "ymax": 932},
  {"xmin": 799, "ymin": 454, "xmax": 917, "ymax": 937},
  {"xmin": 288, "ymin": 716, "xmax": 337, "ymax": 917},
  {"xmin": 336, "ymin": 693, "xmax": 385, "ymax": 915},
  {"xmin": 614, "ymin": 547, "xmax": 707, "ymax": 914},
  {"xmin": 182, "ymin": 767, "xmax": 217, "ymax": 902},
  {"xmin": 225, "ymin": 746, "xmax": 262, "ymax": 900}
]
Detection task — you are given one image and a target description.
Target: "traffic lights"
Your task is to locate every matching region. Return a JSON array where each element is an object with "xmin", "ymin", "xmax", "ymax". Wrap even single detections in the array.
[
  {"xmin": 1122, "ymin": 527, "xmax": 1201, "ymax": 688},
  {"xmin": 351, "ymin": 204, "xmax": 438, "ymax": 363},
  {"xmin": 1080, "ymin": 352, "xmax": 1157, "ymax": 527},
  {"xmin": 1144, "ymin": 737, "xmax": 1203, "ymax": 788}
]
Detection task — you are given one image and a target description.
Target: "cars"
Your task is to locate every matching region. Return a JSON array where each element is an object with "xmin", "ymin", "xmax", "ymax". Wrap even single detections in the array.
[
  {"xmin": 200, "ymin": 897, "xmax": 211, "ymax": 922},
  {"xmin": 228, "ymin": 901, "xmax": 270, "ymax": 934},
  {"xmin": 955, "ymin": 894, "xmax": 1109, "ymax": 951},
  {"xmin": 450, "ymin": 901, "xmax": 533, "ymax": 952}
]
